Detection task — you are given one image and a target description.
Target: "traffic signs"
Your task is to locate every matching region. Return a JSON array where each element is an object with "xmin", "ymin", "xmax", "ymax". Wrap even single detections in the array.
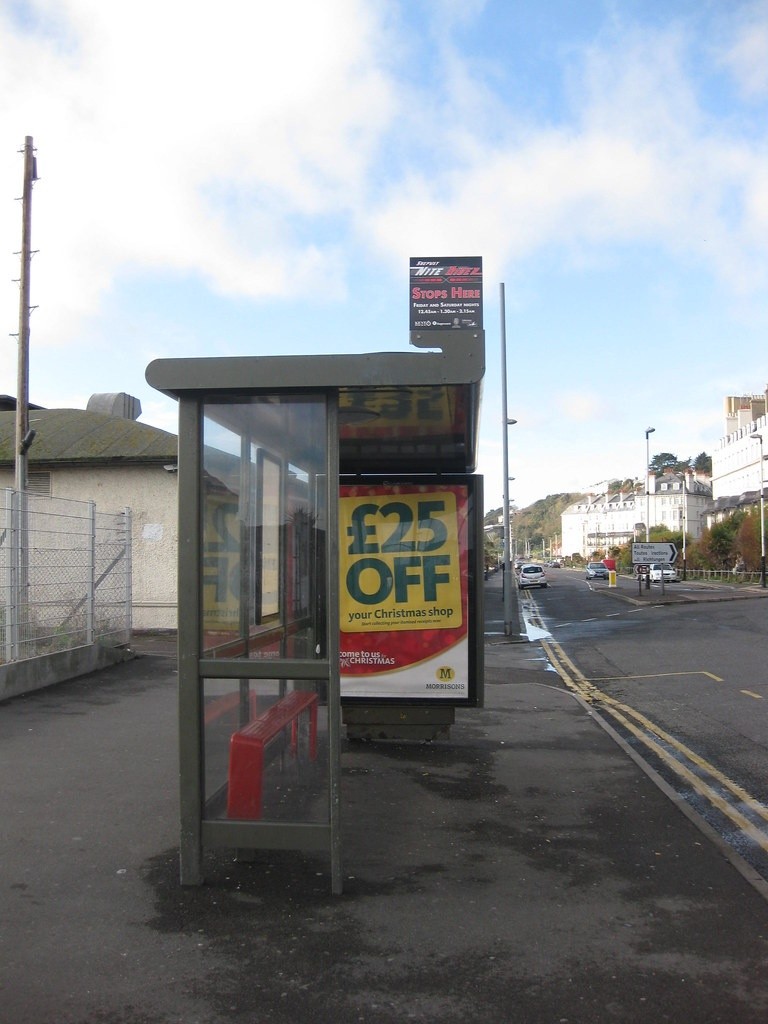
[{"xmin": 630, "ymin": 541, "xmax": 679, "ymax": 565}]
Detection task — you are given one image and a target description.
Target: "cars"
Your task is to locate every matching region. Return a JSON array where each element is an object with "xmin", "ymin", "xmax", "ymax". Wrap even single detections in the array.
[
  {"xmin": 544, "ymin": 560, "xmax": 561, "ymax": 568},
  {"xmin": 517, "ymin": 564, "xmax": 548, "ymax": 589},
  {"xmin": 585, "ymin": 561, "xmax": 611, "ymax": 580},
  {"xmin": 646, "ymin": 563, "xmax": 677, "ymax": 583}
]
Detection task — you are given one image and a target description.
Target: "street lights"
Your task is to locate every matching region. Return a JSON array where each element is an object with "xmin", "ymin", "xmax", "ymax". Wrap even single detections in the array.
[
  {"xmin": 750, "ymin": 434, "xmax": 766, "ymax": 587},
  {"xmin": 644, "ymin": 426, "xmax": 655, "ymax": 542}
]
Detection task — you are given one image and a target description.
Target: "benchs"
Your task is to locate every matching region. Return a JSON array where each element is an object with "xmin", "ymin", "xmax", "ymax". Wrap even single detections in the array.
[{"xmin": 227, "ymin": 688, "xmax": 321, "ymax": 824}]
